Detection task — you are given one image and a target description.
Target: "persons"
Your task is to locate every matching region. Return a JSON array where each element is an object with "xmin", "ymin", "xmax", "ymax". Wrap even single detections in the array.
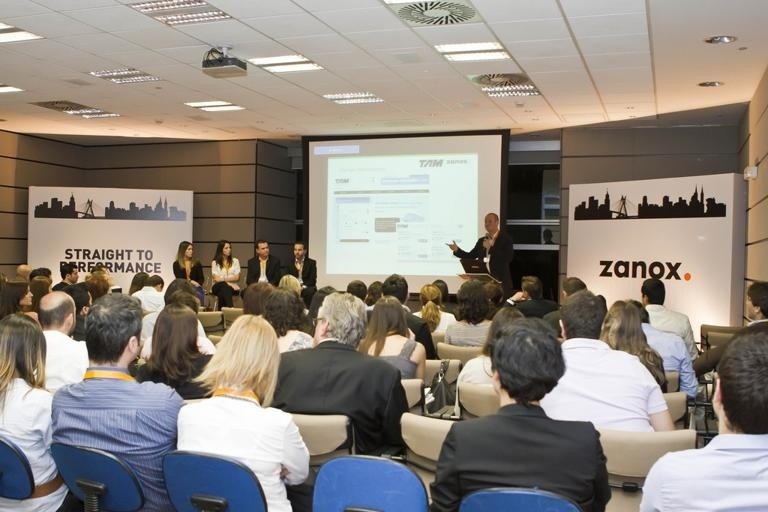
[
  {"xmin": 1, "ymin": 261, "xmax": 768, "ymax": 512},
  {"xmin": 538, "ymin": 228, "xmax": 560, "ymax": 303},
  {"xmin": 446, "ymin": 214, "xmax": 515, "ymax": 308},
  {"xmin": 211, "ymin": 239, "xmax": 242, "ymax": 312},
  {"xmin": 242, "ymin": 239, "xmax": 283, "ymax": 297},
  {"xmin": 173, "ymin": 241, "xmax": 208, "ymax": 307},
  {"xmin": 285, "ymin": 242, "xmax": 318, "ymax": 311}
]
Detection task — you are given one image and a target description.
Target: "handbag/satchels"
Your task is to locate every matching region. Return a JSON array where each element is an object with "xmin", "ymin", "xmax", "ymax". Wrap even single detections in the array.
[{"xmin": 426, "ymin": 372, "xmax": 456, "ymax": 414}]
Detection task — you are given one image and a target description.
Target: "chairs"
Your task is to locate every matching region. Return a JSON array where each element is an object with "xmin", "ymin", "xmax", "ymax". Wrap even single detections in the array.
[
  {"xmin": 456, "ymin": 485, "xmax": 581, "ymax": 512},
  {"xmin": 397, "ymin": 331, "xmax": 500, "ymax": 424},
  {"xmin": 289, "ymin": 407, "xmax": 456, "ymax": 504},
  {"xmin": 197, "ymin": 306, "xmax": 245, "ymax": 345},
  {"xmin": 50, "ymin": 442, "xmax": 147, "ymax": 512},
  {"xmin": 0, "ymin": 435, "xmax": 35, "ymax": 501},
  {"xmin": 311, "ymin": 456, "xmax": 428, "ymax": 512},
  {"xmin": 161, "ymin": 450, "xmax": 269, "ymax": 511},
  {"xmin": 596, "ymin": 321, "xmax": 744, "ymax": 512}
]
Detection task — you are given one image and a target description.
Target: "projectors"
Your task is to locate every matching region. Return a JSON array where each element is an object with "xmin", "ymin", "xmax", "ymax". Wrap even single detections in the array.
[{"xmin": 202, "ymin": 56, "xmax": 247, "ymax": 79}]
